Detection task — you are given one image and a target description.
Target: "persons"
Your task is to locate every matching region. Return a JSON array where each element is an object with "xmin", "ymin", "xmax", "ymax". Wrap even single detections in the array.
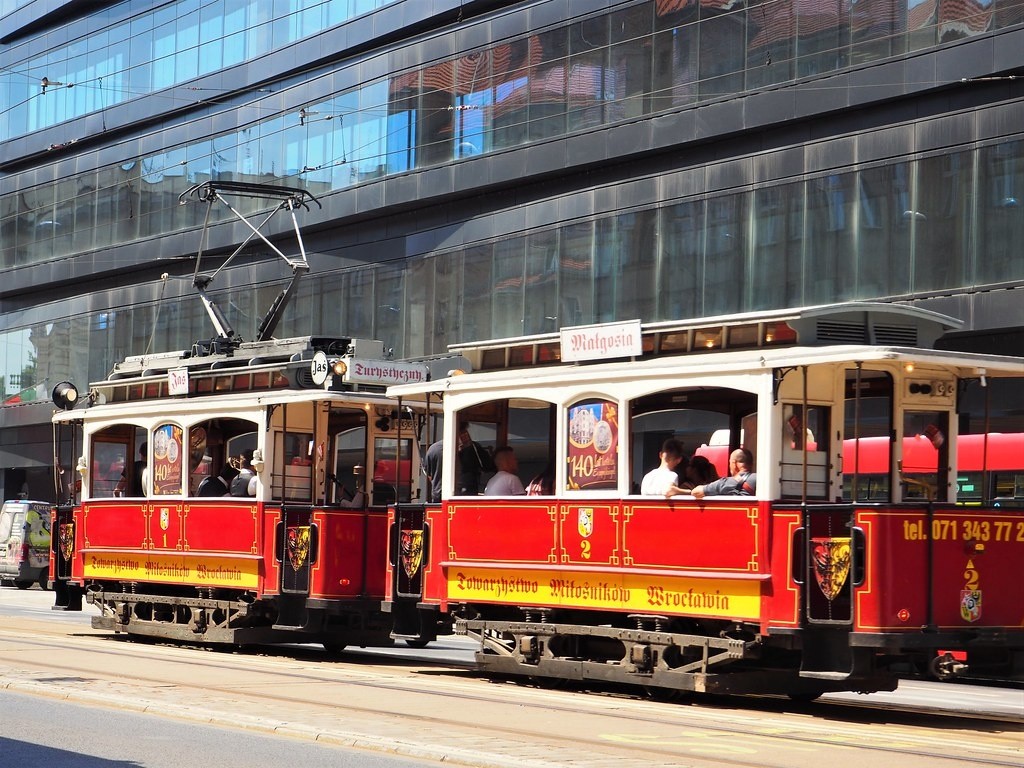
[
  {"xmin": 525, "ymin": 456, "xmax": 556, "ymax": 495},
  {"xmin": 194, "ymin": 457, "xmax": 240, "ymax": 497},
  {"xmin": 424, "ymin": 421, "xmax": 494, "ymax": 503},
  {"xmin": 691, "ymin": 448, "xmax": 756, "ymax": 499},
  {"xmin": 639, "ymin": 438, "xmax": 691, "ymax": 497},
  {"xmin": 483, "ymin": 446, "xmax": 526, "ymax": 495},
  {"xmin": 335, "ymin": 461, "xmax": 396, "ymax": 509},
  {"xmin": 113, "ymin": 442, "xmax": 148, "ymax": 497},
  {"xmin": 680, "ymin": 456, "xmax": 720, "ymax": 490},
  {"xmin": 229, "ymin": 449, "xmax": 257, "ymax": 498}
]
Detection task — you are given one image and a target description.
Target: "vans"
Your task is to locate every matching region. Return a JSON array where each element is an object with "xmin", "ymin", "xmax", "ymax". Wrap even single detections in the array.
[{"xmin": 0, "ymin": 499, "xmax": 61, "ymax": 591}]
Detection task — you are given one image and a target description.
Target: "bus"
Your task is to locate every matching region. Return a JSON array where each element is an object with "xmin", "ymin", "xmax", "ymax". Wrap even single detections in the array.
[{"xmin": 45, "ymin": 179, "xmax": 1024, "ymax": 703}]
